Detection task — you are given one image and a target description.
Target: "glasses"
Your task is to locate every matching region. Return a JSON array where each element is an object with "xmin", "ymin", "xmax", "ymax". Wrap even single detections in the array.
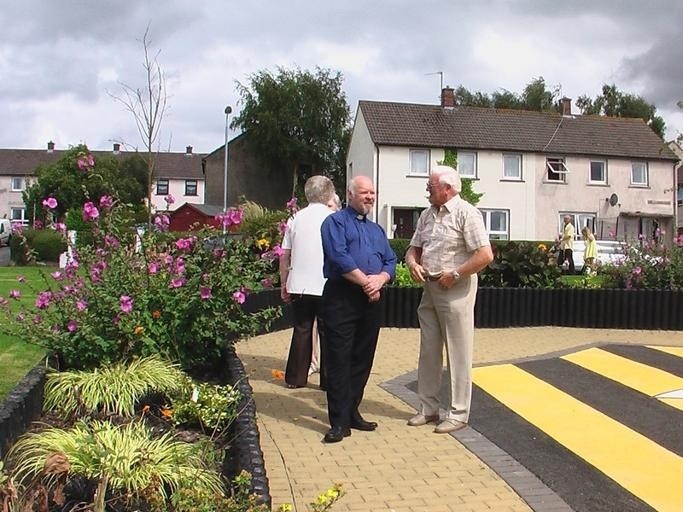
[{"xmin": 426, "ymin": 181, "xmax": 438, "ymax": 187}]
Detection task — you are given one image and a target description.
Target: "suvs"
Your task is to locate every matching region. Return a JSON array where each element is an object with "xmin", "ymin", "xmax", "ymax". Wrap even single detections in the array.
[{"xmin": 547, "ymin": 240, "xmax": 670, "ymax": 281}]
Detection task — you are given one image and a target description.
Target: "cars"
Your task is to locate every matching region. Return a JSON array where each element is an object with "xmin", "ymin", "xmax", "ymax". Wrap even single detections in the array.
[{"xmin": 203, "ymin": 234, "xmax": 222, "ymax": 245}]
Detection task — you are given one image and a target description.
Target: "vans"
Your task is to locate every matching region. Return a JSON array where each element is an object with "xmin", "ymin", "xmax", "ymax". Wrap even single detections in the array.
[{"xmin": 0, "ymin": 218, "xmax": 14, "ymax": 247}]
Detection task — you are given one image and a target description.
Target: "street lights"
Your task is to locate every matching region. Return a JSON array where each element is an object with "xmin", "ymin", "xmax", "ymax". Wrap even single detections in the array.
[{"xmin": 222, "ymin": 105, "xmax": 233, "ymax": 246}]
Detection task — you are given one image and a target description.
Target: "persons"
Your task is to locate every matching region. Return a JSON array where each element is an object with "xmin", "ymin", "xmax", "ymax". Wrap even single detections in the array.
[
  {"xmin": 318, "ymin": 175, "xmax": 398, "ymax": 442},
  {"xmin": 402, "ymin": 165, "xmax": 496, "ymax": 434},
  {"xmin": 553, "ymin": 214, "xmax": 577, "ymax": 275},
  {"xmin": 306, "ymin": 192, "xmax": 345, "ymax": 378},
  {"xmin": 580, "ymin": 227, "xmax": 597, "ymax": 276},
  {"xmin": 278, "ymin": 175, "xmax": 334, "ymax": 391}
]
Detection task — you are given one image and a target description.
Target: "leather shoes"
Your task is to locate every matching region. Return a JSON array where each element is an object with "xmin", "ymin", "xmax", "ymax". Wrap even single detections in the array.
[
  {"xmin": 408, "ymin": 413, "xmax": 439, "ymax": 426},
  {"xmin": 324, "ymin": 425, "xmax": 352, "ymax": 443},
  {"xmin": 435, "ymin": 418, "xmax": 468, "ymax": 433},
  {"xmin": 350, "ymin": 418, "xmax": 378, "ymax": 431}
]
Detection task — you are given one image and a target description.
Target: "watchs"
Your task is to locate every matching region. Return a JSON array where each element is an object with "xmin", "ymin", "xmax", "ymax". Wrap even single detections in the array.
[{"xmin": 452, "ymin": 269, "xmax": 463, "ymax": 286}]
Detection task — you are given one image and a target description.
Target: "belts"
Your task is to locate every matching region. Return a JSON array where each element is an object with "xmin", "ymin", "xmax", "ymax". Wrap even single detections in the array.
[{"xmin": 422, "ymin": 276, "xmax": 438, "ymax": 283}]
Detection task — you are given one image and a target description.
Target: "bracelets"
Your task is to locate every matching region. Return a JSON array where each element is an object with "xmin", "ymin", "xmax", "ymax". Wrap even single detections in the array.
[{"xmin": 280, "ymin": 282, "xmax": 287, "ymax": 287}]
